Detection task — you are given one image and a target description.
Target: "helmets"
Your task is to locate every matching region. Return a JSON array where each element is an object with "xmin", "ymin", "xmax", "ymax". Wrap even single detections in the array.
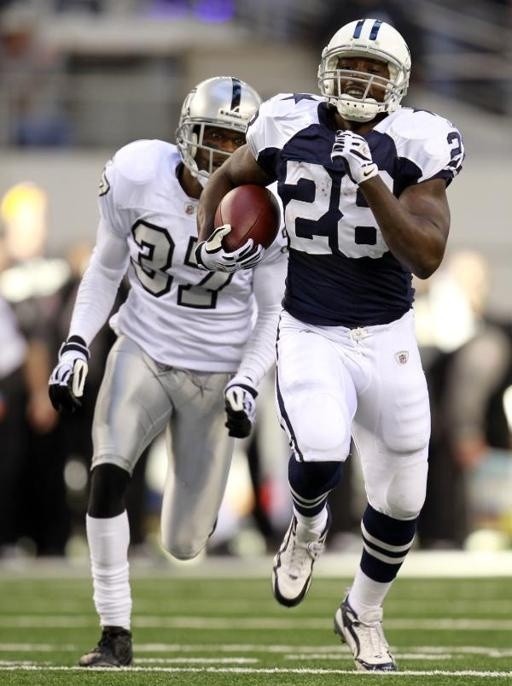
[
  {"xmin": 317, "ymin": 18, "xmax": 411, "ymax": 123},
  {"xmin": 174, "ymin": 77, "xmax": 261, "ymax": 189}
]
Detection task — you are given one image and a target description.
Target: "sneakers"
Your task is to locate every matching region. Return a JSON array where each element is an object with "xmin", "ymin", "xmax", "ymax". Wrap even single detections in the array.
[
  {"xmin": 79, "ymin": 626, "xmax": 132, "ymax": 665},
  {"xmin": 272, "ymin": 504, "xmax": 332, "ymax": 606},
  {"xmin": 334, "ymin": 594, "xmax": 396, "ymax": 671}
]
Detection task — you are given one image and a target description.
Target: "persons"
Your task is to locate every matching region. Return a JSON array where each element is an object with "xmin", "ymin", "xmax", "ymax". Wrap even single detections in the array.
[
  {"xmin": 0, "ymin": 219, "xmax": 512, "ymax": 561},
  {"xmin": 46, "ymin": 73, "xmax": 286, "ymax": 671},
  {"xmin": 194, "ymin": 17, "xmax": 466, "ymax": 668}
]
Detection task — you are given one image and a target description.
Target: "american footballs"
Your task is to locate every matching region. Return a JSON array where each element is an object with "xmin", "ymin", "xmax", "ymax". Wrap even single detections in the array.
[{"xmin": 212, "ymin": 184, "xmax": 281, "ymax": 253}]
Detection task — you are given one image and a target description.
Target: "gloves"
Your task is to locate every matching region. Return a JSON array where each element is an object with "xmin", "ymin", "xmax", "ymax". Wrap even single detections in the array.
[
  {"xmin": 330, "ymin": 130, "xmax": 379, "ymax": 184},
  {"xmin": 225, "ymin": 385, "xmax": 257, "ymax": 438},
  {"xmin": 48, "ymin": 342, "xmax": 91, "ymax": 411},
  {"xmin": 194, "ymin": 224, "xmax": 265, "ymax": 273}
]
